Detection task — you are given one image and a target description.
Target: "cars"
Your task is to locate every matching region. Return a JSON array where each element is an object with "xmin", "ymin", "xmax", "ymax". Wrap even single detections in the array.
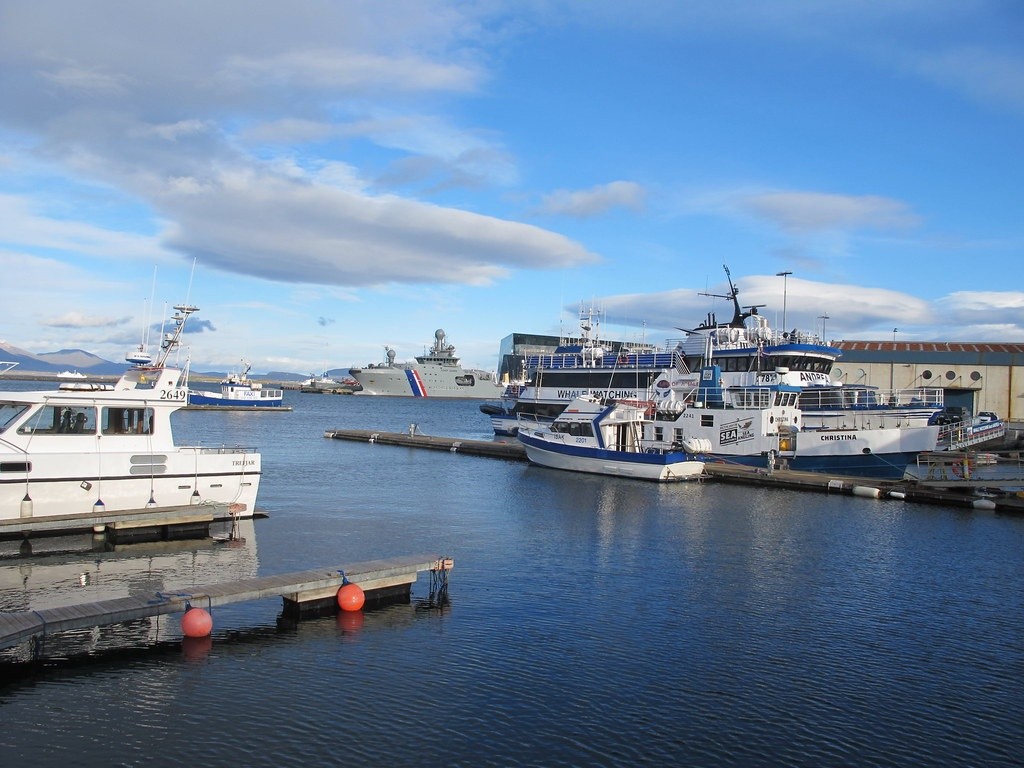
[{"xmin": 975, "ymin": 411, "xmax": 1000, "ymax": 423}]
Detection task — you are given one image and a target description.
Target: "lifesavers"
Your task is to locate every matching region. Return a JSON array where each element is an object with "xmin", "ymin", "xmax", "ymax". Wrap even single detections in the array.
[
  {"xmin": 618, "ymin": 355, "xmax": 629, "ymax": 364},
  {"xmin": 953, "ymin": 459, "xmax": 974, "ymax": 478}
]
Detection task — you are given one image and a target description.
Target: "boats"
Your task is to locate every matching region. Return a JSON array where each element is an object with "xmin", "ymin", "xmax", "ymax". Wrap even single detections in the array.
[
  {"xmin": 455, "ymin": 378, "xmax": 472, "ymax": 384},
  {"xmin": 300, "ymin": 378, "xmax": 339, "ymax": 385},
  {"xmin": 178, "ymin": 357, "xmax": 283, "ymax": 407},
  {"xmin": 56, "ymin": 370, "xmax": 87, "ymax": 378},
  {"xmin": 480, "ymin": 264, "xmax": 943, "ymax": 484},
  {"xmin": 0, "ymin": 256, "xmax": 262, "ymax": 520}
]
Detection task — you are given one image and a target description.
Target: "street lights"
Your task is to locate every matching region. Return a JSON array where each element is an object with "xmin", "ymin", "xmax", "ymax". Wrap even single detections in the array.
[{"xmin": 776, "ymin": 271, "xmax": 792, "ymax": 332}]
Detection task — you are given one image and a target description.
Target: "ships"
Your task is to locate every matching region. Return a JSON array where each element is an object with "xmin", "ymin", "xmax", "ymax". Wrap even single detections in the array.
[{"xmin": 349, "ymin": 329, "xmax": 505, "ymax": 399}]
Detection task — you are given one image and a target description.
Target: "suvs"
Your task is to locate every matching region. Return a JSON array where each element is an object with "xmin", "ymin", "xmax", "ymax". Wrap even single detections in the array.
[{"xmin": 941, "ymin": 406, "xmax": 971, "ymax": 425}]
